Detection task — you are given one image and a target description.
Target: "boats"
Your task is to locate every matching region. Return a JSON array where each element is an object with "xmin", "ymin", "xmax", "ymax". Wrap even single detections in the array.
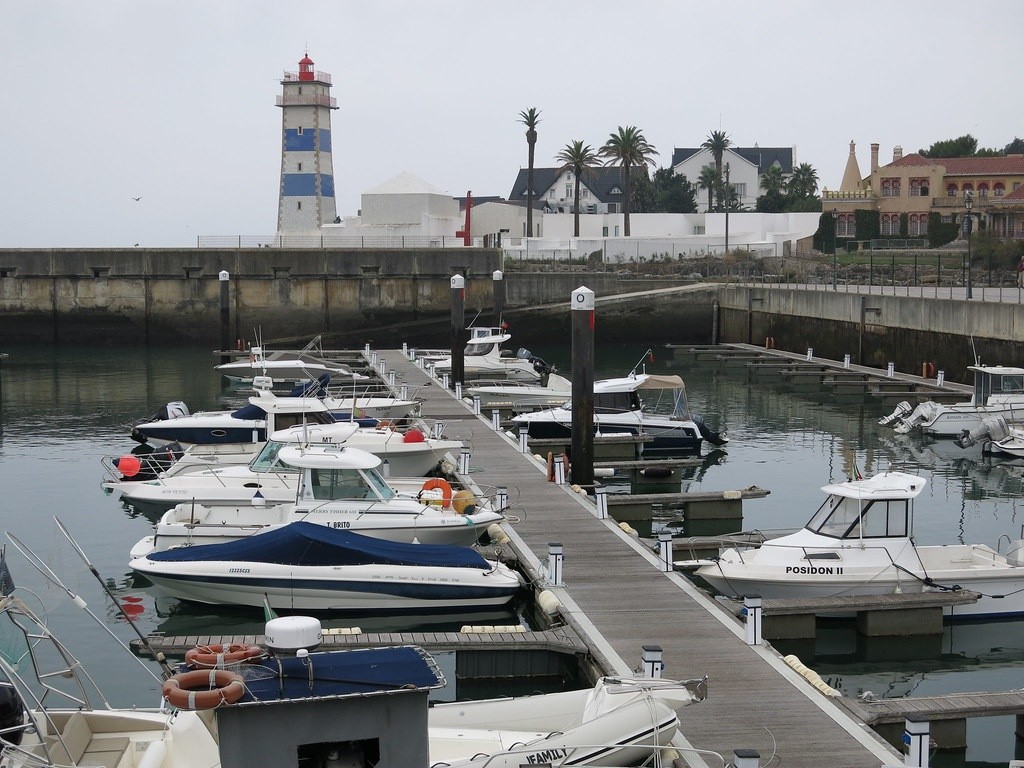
[
  {"xmin": 413, "ymin": 323, "xmax": 573, "ymax": 407},
  {"xmin": 881, "ymin": 366, "xmax": 1024, "ymax": 462},
  {"xmin": 694, "ymin": 460, "xmax": 1024, "ymax": 620},
  {"xmin": 509, "ymin": 369, "xmax": 707, "ymax": 458},
  {"xmin": 1, "ymin": 344, "xmax": 710, "ymax": 768}
]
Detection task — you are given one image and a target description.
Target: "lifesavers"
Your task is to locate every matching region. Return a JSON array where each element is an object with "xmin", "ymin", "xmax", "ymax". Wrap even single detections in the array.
[
  {"xmin": 184, "ymin": 643, "xmax": 261, "ymax": 671},
  {"xmin": 422, "ymin": 479, "xmax": 451, "ymax": 508},
  {"xmin": 163, "ymin": 669, "xmax": 246, "ymax": 709},
  {"xmin": 375, "ymin": 421, "xmax": 396, "ymax": 434}
]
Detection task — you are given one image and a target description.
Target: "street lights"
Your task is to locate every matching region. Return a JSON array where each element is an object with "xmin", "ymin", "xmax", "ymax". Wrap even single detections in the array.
[
  {"xmin": 964, "ymin": 191, "xmax": 974, "ymax": 296},
  {"xmin": 832, "ymin": 209, "xmax": 840, "ymax": 292}
]
